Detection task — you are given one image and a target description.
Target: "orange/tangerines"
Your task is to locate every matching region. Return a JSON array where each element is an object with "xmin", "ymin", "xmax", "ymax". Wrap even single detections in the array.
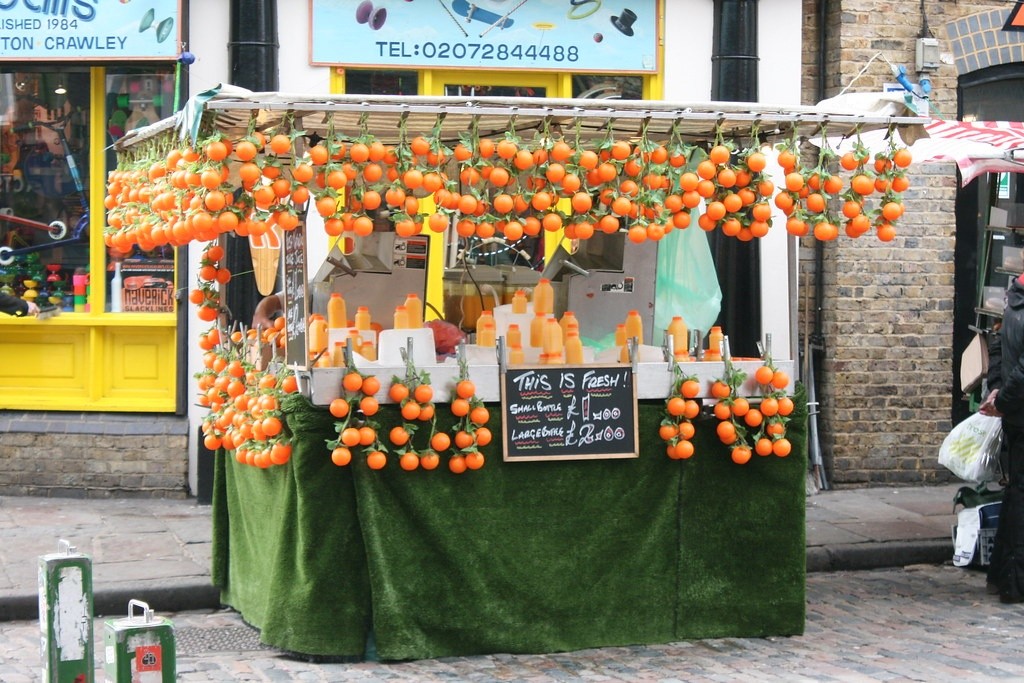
[
  {"xmin": 660, "ymin": 367, "xmax": 794, "ymax": 464},
  {"xmin": 330, "ymin": 374, "xmax": 492, "ymax": 473},
  {"xmin": 104, "ymin": 133, "xmax": 912, "ymax": 255},
  {"xmin": 231, "ymin": 313, "xmax": 387, "ymax": 368},
  {"xmin": 191, "ymin": 249, "xmax": 299, "ymax": 470}
]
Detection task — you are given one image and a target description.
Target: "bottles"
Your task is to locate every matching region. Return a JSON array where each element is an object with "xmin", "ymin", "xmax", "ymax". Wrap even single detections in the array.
[
  {"xmin": 702, "ymin": 327, "xmax": 724, "ymax": 361},
  {"xmin": 616, "ymin": 310, "xmax": 643, "ymax": 362},
  {"xmin": 669, "ymin": 316, "xmax": 690, "ymax": 361},
  {"xmin": 308, "ymin": 293, "xmax": 376, "ymax": 369},
  {"xmin": 476, "ymin": 310, "xmax": 497, "ymax": 346},
  {"xmin": 504, "ymin": 278, "xmax": 583, "ymax": 366},
  {"xmin": 394, "ymin": 294, "xmax": 423, "ymax": 329},
  {"xmin": 111, "ymin": 262, "xmax": 123, "ymax": 312}
]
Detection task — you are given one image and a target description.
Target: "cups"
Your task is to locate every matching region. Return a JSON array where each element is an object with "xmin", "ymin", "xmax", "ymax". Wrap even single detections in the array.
[{"xmin": 73, "ymin": 273, "xmax": 90, "ymax": 312}]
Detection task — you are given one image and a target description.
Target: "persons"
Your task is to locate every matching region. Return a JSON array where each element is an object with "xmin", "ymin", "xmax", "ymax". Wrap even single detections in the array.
[
  {"xmin": 252, "ymin": 291, "xmax": 285, "ymax": 329},
  {"xmin": 979, "ymin": 273, "xmax": 1024, "ymax": 603}
]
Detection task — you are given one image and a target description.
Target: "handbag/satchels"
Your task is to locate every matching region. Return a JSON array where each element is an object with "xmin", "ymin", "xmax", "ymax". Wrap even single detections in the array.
[
  {"xmin": 952, "ymin": 479, "xmax": 1006, "ymax": 525},
  {"xmin": 938, "ymin": 412, "xmax": 1004, "ymax": 484}
]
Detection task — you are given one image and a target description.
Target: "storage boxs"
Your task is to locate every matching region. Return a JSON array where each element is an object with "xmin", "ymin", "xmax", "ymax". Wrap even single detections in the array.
[{"xmin": 270, "ymin": 347, "xmax": 796, "ymax": 405}]
